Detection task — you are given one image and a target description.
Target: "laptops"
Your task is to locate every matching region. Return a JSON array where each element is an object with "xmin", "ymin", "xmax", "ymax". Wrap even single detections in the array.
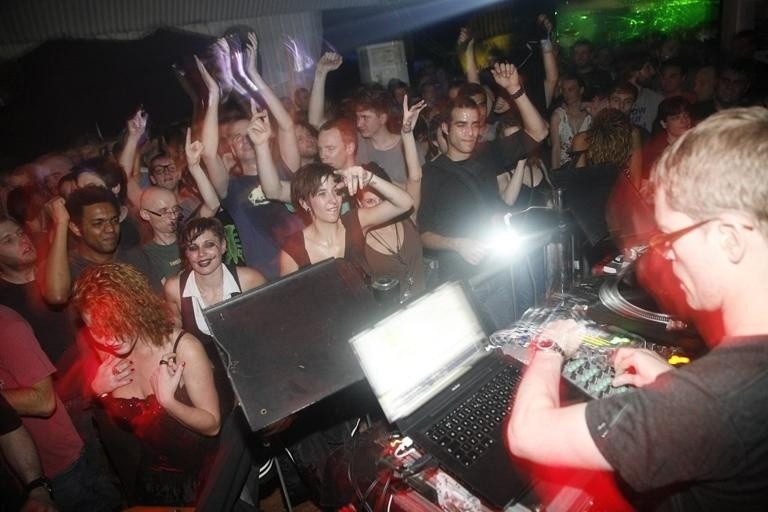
[{"xmin": 347, "ymin": 271, "xmax": 595, "ymax": 512}]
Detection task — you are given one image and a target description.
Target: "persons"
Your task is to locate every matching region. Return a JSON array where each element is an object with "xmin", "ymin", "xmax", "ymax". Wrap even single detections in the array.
[
  {"xmin": 754, "ymin": 48, "xmax": 768, "ymax": 106},
  {"xmin": 0, "ymin": 392, "xmax": 57, "ymax": 512},
  {"xmin": 119, "ymin": 107, "xmax": 199, "ymax": 215},
  {"xmin": 75, "ymin": 157, "xmax": 124, "ymax": 200},
  {"xmin": 686, "ymin": 63, "xmax": 717, "ymax": 106},
  {"xmin": 420, "ymin": 81, "xmax": 437, "ymax": 101},
  {"xmin": 387, "ymin": 78, "xmax": 411, "ymax": 134},
  {"xmin": 658, "ymin": 34, "xmax": 684, "ymax": 60},
  {"xmin": 295, "ymin": 88, "xmax": 311, "ymax": 124},
  {"xmin": 568, "ymin": 35, "xmax": 605, "ymax": 75},
  {"xmin": 294, "ymin": 120, "xmax": 321, "ymax": 167},
  {"xmin": 611, "ymin": 81, "xmax": 651, "ymax": 143},
  {"xmin": 280, "ymin": 97, "xmax": 293, "ymax": 114},
  {"xmin": 305, "ymin": 51, "xmax": 428, "ymax": 310},
  {"xmin": 0, "ymin": 305, "xmax": 87, "ymax": 512},
  {"xmin": 247, "ymin": 98, "xmax": 393, "ymax": 217},
  {"xmin": 37, "ymin": 183, "xmax": 123, "ymax": 306},
  {"xmin": 551, "ymin": 76, "xmax": 596, "ymax": 169},
  {"xmin": 659, "ymin": 57, "xmax": 686, "ymax": 95},
  {"xmin": 59, "ymin": 263, "xmax": 220, "ymax": 512},
  {"xmin": 572, "ymin": 76, "xmax": 643, "ymax": 272},
  {"xmin": 420, "ymin": 107, "xmax": 450, "ymax": 175},
  {"xmin": 280, "ymin": 161, "xmax": 414, "ymax": 300},
  {"xmin": 0, "ymin": 217, "xmax": 74, "ymax": 383},
  {"xmin": 626, "ymin": 57, "xmax": 656, "ymax": 85},
  {"xmin": 718, "ymin": 63, "xmax": 754, "ymax": 106},
  {"xmin": 57, "ymin": 174, "xmax": 80, "ymax": 202},
  {"xmin": 731, "ymin": 28, "xmax": 756, "ymax": 62},
  {"xmin": 506, "ymin": 106, "xmax": 768, "ymax": 512},
  {"xmin": 122, "ymin": 121, "xmax": 162, "ymax": 167},
  {"xmin": 163, "ymin": 216, "xmax": 292, "ymax": 437},
  {"xmin": 164, "ymin": 129, "xmax": 190, "ymax": 165},
  {"xmin": 138, "ymin": 126, "xmax": 221, "ymax": 283},
  {"xmin": 642, "ymin": 95, "xmax": 691, "ymax": 170},
  {"xmin": 416, "ymin": 98, "xmax": 528, "ymax": 335},
  {"xmin": 453, "ymin": 16, "xmax": 559, "ymax": 124},
  {"xmin": 194, "ymin": 28, "xmax": 295, "ymax": 284},
  {"xmin": 496, "ymin": 116, "xmax": 554, "ymax": 314},
  {"xmin": 457, "ymin": 80, "xmax": 499, "ymax": 143},
  {"xmin": 5, "ymin": 186, "xmax": 52, "ymax": 240},
  {"xmin": 447, "ymin": 83, "xmax": 460, "ymax": 99},
  {"xmin": 596, "ymin": 44, "xmax": 615, "ymax": 75}
]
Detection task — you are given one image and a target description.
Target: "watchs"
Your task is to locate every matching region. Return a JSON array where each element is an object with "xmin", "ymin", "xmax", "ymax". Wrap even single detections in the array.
[
  {"xmin": 535, "ymin": 339, "xmax": 566, "ymax": 357},
  {"xmin": 25, "ymin": 478, "xmax": 55, "ymax": 498}
]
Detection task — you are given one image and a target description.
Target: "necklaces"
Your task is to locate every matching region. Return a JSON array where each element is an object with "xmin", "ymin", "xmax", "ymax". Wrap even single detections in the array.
[{"xmin": 369, "ymin": 220, "xmax": 414, "ymax": 287}]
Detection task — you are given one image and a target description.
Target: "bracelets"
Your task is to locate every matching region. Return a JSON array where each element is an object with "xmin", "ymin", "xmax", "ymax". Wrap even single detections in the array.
[{"xmin": 367, "ymin": 173, "xmax": 375, "ymax": 186}]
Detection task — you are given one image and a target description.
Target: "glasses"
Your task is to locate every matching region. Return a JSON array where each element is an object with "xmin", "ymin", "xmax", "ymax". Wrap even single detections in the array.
[
  {"xmin": 150, "ymin": 163, "xmax": 177, "ymax": 176},
  {"xmin": 650, "ymin": 216, "xmax": 753, "ymax": 255},
  {"xmin": 143, "ymin": 205, "xmax": 184, "ymax": 218}
]
